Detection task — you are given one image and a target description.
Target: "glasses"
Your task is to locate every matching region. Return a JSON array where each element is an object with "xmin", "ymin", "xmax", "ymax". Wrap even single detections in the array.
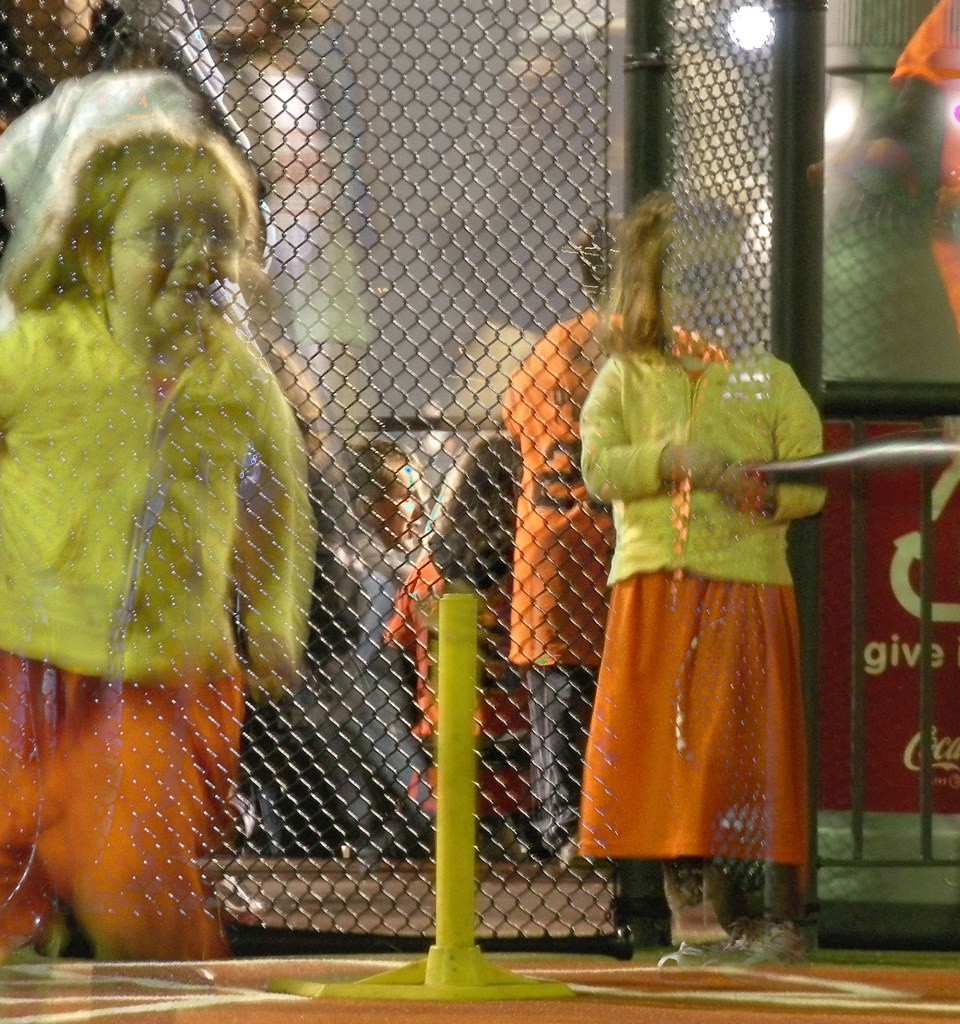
[{"xmin": 109, "ymin": 221, "xmax": 258, "ymax": 261}]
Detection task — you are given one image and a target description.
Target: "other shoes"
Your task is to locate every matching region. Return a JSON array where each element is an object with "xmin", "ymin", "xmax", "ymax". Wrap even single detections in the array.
[{"xmin": 659, "ymin": 920, "xmax": 815, "ymax": 969}]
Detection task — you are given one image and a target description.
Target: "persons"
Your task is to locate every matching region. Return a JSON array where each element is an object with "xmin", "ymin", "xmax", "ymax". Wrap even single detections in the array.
[
  {"xmin": 578, "ymin": 195, "xmax": 829, "ymax": 966},
  {"xmin": 803, "ymin": 0, "xmax": 959, "ymax": 353},
  {"xmin": 0, "ymin": 0, "xmax": 828, "ymax": 961},
  {"xmin": 0, "ymin": 70, "xmax": 319, "ymax": 959}
]
